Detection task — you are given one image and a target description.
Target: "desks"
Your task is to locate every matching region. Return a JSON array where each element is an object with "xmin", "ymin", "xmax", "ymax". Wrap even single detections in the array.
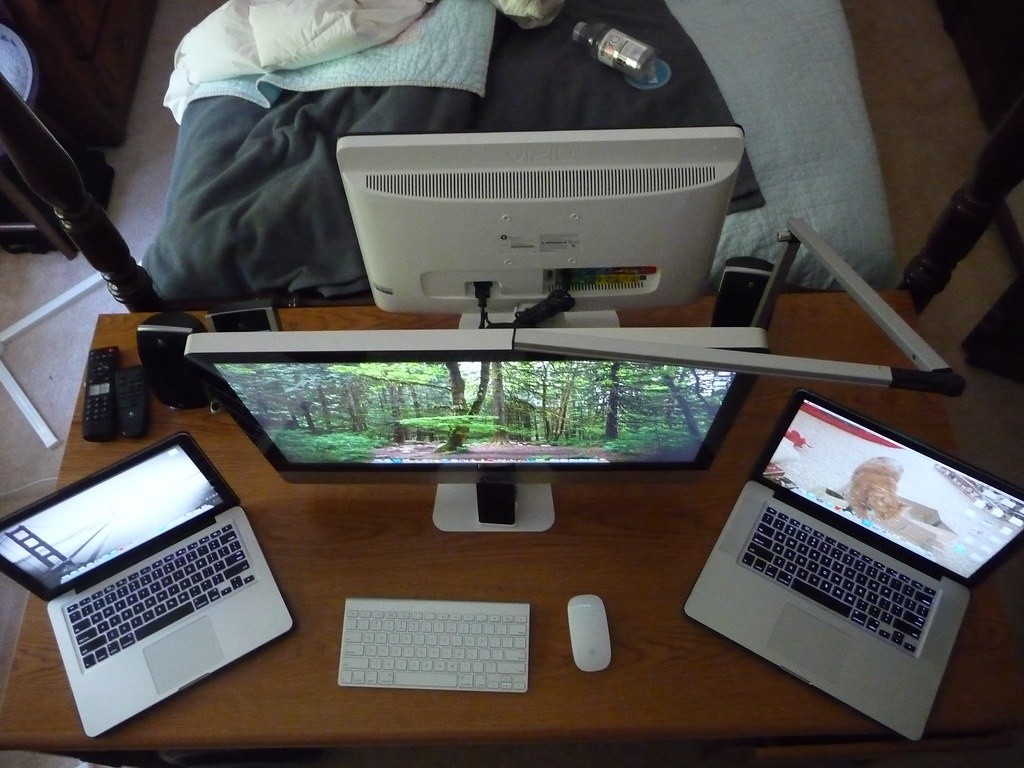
[{"xmin": 1, "ymin": 286, "xmax": 1023, "ymax": 751}]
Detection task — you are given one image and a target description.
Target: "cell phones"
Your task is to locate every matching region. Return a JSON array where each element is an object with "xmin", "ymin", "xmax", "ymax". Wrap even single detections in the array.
[{"xmin": 476, "ymin": 483, "xmax": 515, "ymax": 525}]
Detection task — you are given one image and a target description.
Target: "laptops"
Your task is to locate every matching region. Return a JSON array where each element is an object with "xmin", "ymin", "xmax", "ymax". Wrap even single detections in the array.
[
  {"xmin": 682, "ymin": 388, "xmax": 1024, "ymax": 741},
  {"xmin": 1, "ymin": 429, "xmax": 290, "ymax": 738}
]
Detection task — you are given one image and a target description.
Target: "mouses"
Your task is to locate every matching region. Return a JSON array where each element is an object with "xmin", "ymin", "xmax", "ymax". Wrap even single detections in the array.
[{"xmin": 567, "ymin": 594, "xmax": 611, "ymax": 672}]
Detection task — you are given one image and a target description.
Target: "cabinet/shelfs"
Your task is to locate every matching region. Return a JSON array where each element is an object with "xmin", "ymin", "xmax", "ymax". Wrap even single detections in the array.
[{"xmin": 0, "ymin": 0, "xmax": 155, "ymax": 146}]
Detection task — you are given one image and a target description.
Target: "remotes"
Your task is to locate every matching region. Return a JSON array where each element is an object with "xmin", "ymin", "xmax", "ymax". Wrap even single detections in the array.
[{"xmin": 82, "ymin": 344, "xmax": 149, "ymax": 443}]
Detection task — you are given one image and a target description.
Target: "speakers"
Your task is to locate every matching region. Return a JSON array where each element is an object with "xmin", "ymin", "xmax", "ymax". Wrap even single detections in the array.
[
  {"xmin": 709, "ymin": 256, "xmax": 775, "ymax": 329},
  {"xmin": 136, "ymin": 295, "xmax": 283, "ymax": 410}
]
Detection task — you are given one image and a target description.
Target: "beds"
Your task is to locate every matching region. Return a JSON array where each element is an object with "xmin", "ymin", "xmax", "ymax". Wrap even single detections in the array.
[{"xmin": 159, "ymin": 0, "xmax": 900, "ymax": 289}]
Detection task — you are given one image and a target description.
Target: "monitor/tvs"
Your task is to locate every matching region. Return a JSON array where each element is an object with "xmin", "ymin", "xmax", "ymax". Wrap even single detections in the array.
[
  {"xmin": 333, "ymin": 121, "xmax": 747, "ymax": 314},
  {"xmin": 182, "ymin": 328, "xmax": 771, "ymax": 483}
]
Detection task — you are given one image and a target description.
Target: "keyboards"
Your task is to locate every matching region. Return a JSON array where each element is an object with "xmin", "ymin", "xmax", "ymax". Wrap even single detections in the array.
[{"xmin": 337, "ymin": 597, "xmax": 530, "ymax": 694}]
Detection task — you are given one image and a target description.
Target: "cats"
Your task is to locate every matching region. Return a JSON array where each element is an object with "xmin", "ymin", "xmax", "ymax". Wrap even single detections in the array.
[{"xmin": 842, "ymin": 456, "xmax": 910, "ymax": 525}]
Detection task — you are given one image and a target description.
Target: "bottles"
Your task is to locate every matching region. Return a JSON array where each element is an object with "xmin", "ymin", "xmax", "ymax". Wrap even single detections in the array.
[{"xmin": 572, "ymin": 21, "xmax": 660, "ymax": 78}]
